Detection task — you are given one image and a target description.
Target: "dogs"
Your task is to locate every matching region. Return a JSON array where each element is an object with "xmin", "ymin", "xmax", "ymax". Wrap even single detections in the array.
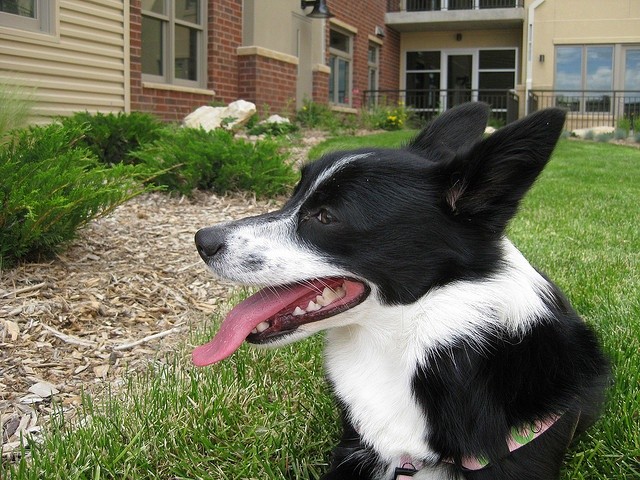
[{"xmin": 191, "ymin": 101, "xmax": 610, "ymax": 479}]
